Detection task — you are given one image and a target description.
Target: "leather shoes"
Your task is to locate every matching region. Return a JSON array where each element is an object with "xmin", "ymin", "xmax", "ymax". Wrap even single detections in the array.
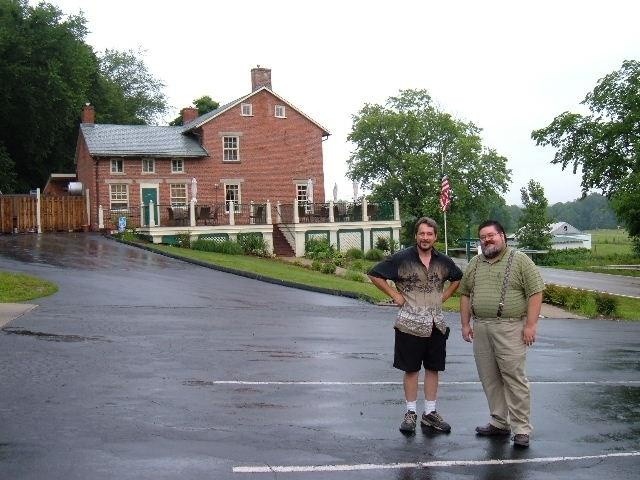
[
  {"xmin": 476, "ymin": 423, "xmax": 511, "ymax": 435},
  {"xmin": 514, "ymin": 434, "xmax": 529, "ymax": 447}
]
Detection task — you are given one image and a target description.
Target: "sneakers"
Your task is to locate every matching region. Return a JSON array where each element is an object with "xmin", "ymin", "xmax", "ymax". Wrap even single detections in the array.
[
  {"xmin": 422, "ymin": 411, "xmax": 451, "ymax": 431},
  {"xmin": 399, "ymin": 410, "xmax": 417, "ymax": 431}
]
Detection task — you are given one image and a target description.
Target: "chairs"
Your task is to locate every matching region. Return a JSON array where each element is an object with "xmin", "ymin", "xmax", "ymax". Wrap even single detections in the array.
[
  {"xmin": 168, "ymin": 206, "xmax": 222, "ymax": 225},
  {"xmin": 249, "ymin": 206, "xmax": 263, "ymax": 223},
  {"xmin": 306, "ymin": 206, "xmax": 352, "ymax": 222}
]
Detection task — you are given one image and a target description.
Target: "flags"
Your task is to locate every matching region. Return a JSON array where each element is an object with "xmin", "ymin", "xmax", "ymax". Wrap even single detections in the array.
[{"xmin": 439, "ymin": 173, "xmax": 450, "ymax": 212}]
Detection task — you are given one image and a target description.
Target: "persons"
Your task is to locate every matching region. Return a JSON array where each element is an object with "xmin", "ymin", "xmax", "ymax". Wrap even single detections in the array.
[
  {"xmin": 457, "ymin": 220, "xmax": 546, "ymax": 447},
  {"xmin": 366, "ymin": 216, "xmax": 464, "ymax": 433}
]
